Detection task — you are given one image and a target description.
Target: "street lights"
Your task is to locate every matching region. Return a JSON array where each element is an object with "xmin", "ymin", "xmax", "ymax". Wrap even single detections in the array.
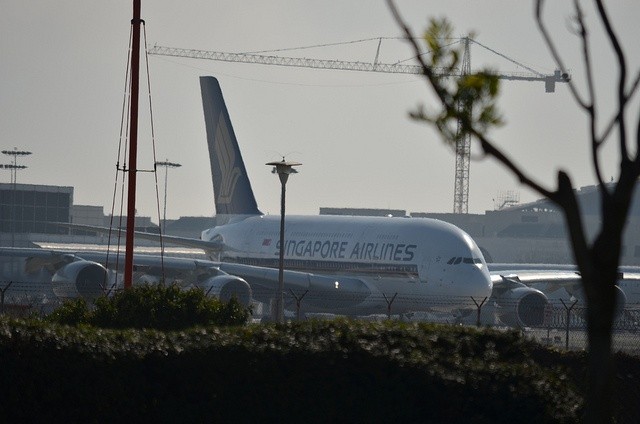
[
  {"xmin": 265, "ymin": 156, "xmax": 304, "ymax": 325},
  {"xmin": 154, "ymin": 158, "xmax": 182, "ymax": 255},
  {"xmin": 1, "ymin": 147, "xmax": 33, "ymax": 285},
  {"xmin": 1, "ymin": 163, "xmax": 27, "ymax": 246}
]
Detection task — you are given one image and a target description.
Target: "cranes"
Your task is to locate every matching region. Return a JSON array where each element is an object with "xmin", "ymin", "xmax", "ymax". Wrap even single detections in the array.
[{"xmin": 147, "ymin": 29, "xmax": 573, "ymax": 215}]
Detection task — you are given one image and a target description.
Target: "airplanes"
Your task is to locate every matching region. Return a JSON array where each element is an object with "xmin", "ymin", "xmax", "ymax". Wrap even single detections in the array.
[
  {"xmin": 1, "ymin": 75, "xmax": 640, "ymax": 331},
  {"xmin": 483, "ymin": 260, "xmax": 640, "ymax": 313}
]
